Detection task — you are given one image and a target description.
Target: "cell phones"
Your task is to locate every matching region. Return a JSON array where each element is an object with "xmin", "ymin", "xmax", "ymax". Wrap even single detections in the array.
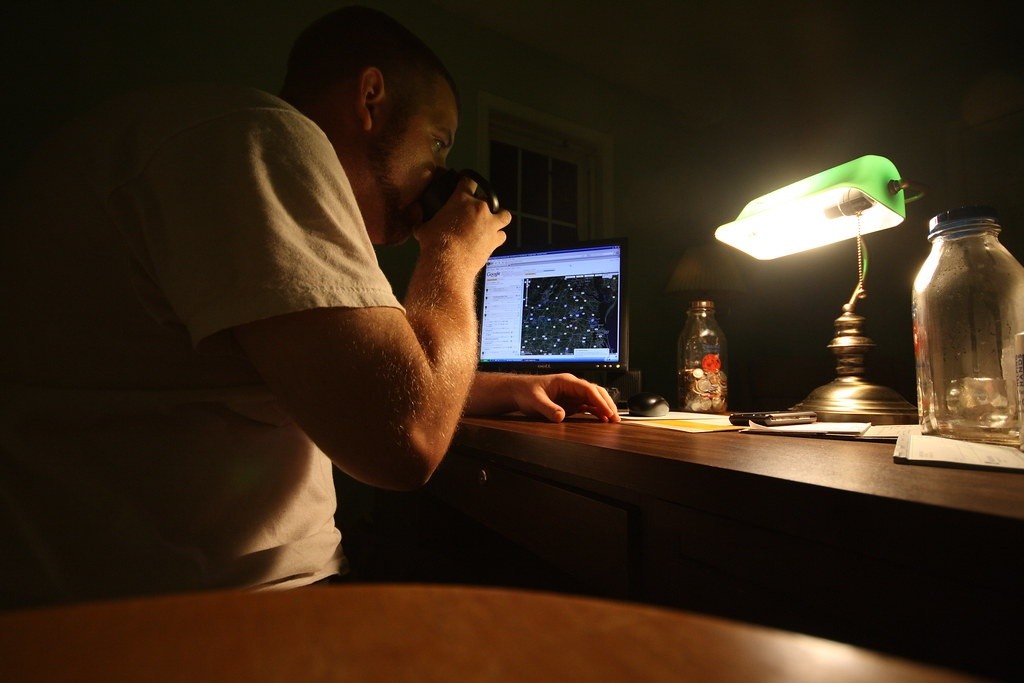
[{"xmin": 729, "ymin": 411, "xmax": 817, "ymax": 426}]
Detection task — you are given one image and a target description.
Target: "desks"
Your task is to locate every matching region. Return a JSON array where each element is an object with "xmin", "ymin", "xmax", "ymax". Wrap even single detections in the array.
[
  {"xmin": 0, "ymin": 585, "xmax": 973, "ymax": 683},
  {"xmin": 425, "ymin": 403, "xmax": 1024, "ymax": 683}
]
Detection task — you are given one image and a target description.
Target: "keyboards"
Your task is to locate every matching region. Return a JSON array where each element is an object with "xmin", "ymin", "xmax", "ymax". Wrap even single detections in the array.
[{"xmin": 604, "ymin": 387, "xmax": 620, "ymax": 403}]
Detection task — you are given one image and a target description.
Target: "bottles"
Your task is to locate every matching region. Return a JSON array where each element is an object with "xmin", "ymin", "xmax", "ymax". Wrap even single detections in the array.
[
  {"xmin": 678, "ymin": 300, "xmax": 728, "ymax": 412},
  {"xmin": 911, "ymin": 205, "xmax": 1024, "ymax": 447}
]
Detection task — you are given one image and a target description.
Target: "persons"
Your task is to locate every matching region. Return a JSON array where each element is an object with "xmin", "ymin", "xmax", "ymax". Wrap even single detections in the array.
[{"xmin": 0, "ymin": 7, "xmax": 619, "ymax": 610}]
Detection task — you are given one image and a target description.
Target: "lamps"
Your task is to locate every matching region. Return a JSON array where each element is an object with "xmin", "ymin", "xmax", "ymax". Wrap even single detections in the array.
[{"xmin": 708, "ymin": 154, "xmax": 924, "ymax": 425}]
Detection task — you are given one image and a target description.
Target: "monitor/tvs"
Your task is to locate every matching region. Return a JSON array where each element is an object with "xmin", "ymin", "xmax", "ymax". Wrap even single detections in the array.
[{"xmin": 475, "ymin": 237, "xmax": 629, "ymax": 374}]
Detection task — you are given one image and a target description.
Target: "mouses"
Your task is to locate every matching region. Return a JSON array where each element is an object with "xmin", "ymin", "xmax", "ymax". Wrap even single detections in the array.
[{"xmin": 629, "ymin": 392, "xmax": 670, "ymax": 417}]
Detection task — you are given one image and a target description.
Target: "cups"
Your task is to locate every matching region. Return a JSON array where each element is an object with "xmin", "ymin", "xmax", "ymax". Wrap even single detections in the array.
[{"xmin": 420, "ymin": 167, "xmax": 499, "ymax": 221}]
